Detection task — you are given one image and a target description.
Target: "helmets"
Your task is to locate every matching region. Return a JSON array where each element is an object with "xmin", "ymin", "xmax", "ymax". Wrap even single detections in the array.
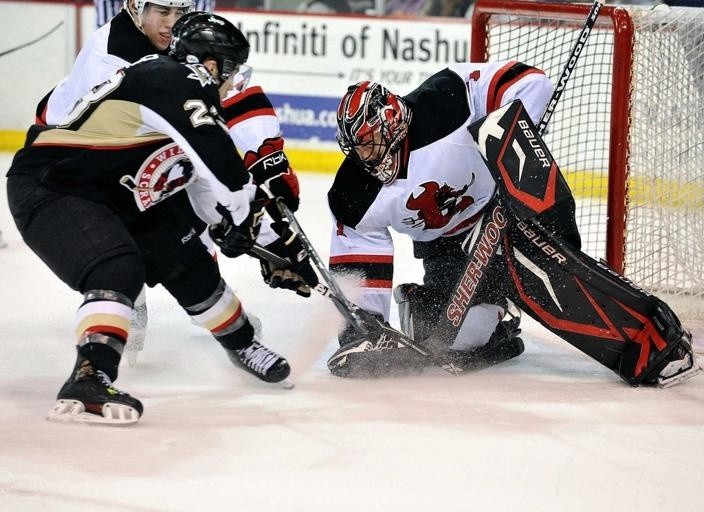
[
  {"xmin": 170, "ymin": 9, "xmax": 252, "ymax": 94},
  {"xmin": 336, "ymin": 80, "xmax": 415, "ymax": 186},
  {"xmin": 121, "ymin": 0, "xmax": 191, "ymax": 27}
]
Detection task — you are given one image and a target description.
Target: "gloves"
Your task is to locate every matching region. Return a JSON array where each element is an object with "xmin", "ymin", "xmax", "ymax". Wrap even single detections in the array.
[
  {"xmin": 206, "ymin": 180, "xmax": 271, "ymax": 257},
  {"xmin": 326, "ymin": 314, "xmax": 425, "ymax": 377},
  {"xmin": 259, "ymin": 234, "xmax": 313, "ymax": 297},
  {"xmin": 244, "ymin": 140, "xmax": 301, "ymax": 218}
]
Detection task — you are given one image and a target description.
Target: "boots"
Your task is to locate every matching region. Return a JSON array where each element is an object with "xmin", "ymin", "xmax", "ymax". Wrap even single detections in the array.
[{"xmin": 55, "ymin": 355, "xmax": 145, "ymax": 421}]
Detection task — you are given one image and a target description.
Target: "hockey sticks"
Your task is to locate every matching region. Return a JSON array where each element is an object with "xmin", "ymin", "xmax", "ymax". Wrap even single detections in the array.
[
  {"xmin": 345, "ymin": 0, "xmax": 605, "ymax": 379},
  {"xmin": 250, "ymin": 244, "xmax": 524, "ymax": 375}
]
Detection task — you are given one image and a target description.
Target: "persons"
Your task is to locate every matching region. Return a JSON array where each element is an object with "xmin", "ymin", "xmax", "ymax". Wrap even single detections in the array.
[
  {"xmin": 4, "ymin": 10, "xmax": 318, "ymax": 420},
  {"xmin": 326, "ymin": 57, "xmax": 692, "ymax": 390},
  {"xmin": 33, "ymin": 0, "xmax": 302, "ymax": 353}
]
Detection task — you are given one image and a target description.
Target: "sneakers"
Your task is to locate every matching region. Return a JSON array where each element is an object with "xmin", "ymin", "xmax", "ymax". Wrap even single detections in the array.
[
  {"xmin": 640, "ymin": 333, "xmax": 694, "ymax": 387},
  {"xmin": 224, "ymin": 339, "xmax": 290, "ymax": 383}
]
